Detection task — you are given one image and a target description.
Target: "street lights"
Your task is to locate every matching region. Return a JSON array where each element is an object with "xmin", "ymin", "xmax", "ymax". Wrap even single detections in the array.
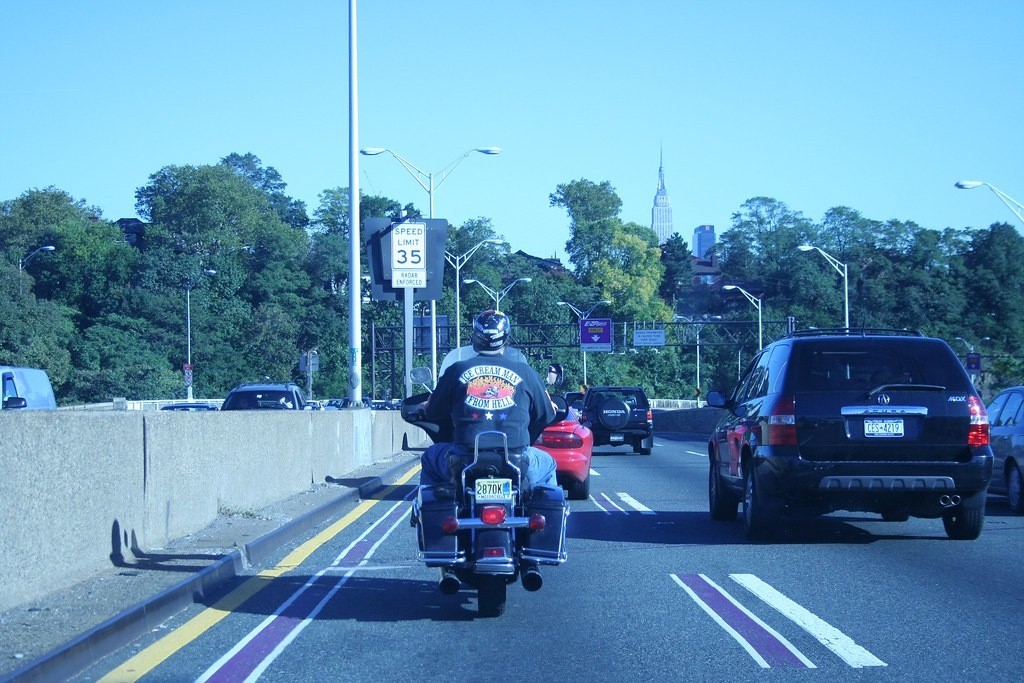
[
  {"xmin": 796, "ymin": 245, "xmax": 850, "ymax": 380},
  {"xmin": 185, "ymin": 270, "xmax": 216, "ymax": 398},
  {"xmin": 556, "ymin": 300, "xmax": 612, "ymax": 390},
  {"xmin": 677, "ymin": 316, "xmax": 723, "ymax": 410},
  {"xmin": 17, "ymin": 246, "xmax": 56, "ymax": 296},
  {"xmin": 721, "ymin": 285, "xmax": 764, "ymax": 350},
  {"xmin": 956, "ymin": 337, "xmax": 991, "ymax": 383},
  {"xmin": 361, "ymin": 146, "xmax": 534, "ymax": 407}
]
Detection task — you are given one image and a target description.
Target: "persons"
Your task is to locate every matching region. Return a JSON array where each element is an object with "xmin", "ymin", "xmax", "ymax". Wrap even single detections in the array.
[{"xmin": 418, "ymin": 309, "xmax": 559, "ymax": 490}]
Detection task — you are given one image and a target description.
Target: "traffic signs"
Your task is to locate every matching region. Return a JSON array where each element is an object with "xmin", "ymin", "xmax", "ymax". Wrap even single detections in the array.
[{"xmin": 580, "ymin": 318, "xmax": 616, "ymax": 351}]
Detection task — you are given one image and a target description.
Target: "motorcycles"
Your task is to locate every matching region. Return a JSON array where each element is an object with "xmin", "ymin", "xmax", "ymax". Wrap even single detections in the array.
[{"xmin": 400, "ymin": 363, "xmax": 570, "ymax": 617}]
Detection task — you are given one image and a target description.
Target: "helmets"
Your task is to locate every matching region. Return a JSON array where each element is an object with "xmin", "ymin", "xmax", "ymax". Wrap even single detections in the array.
[{"xmin": 471, "ymin": 310, "xmax": 512, "ymax": 355}]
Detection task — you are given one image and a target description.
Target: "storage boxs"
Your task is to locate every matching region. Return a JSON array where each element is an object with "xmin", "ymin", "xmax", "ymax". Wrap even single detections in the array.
[
  {"xmin": 522, "ymin": 485, "xmax": 572, "ymax": 567},
  {"xmin": 411, "ymin": 479, "xmax": 466, "ymax": 570}
]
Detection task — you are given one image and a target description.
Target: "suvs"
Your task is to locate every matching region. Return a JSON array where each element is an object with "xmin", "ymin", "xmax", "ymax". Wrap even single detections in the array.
[
  {"xmin": 706, "ymin": 331, "xmax": 997, "ymax": 541},
  {"xmin": 222, "ymin": 376, "xmax": 310, "ymax": 411},
  {"xmin": 566, "ymin": 386, "xmax": 654, "ymax": 457}
]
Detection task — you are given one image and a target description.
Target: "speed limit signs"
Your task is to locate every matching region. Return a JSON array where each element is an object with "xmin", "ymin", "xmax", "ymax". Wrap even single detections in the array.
[{"xmin": 392, "ymin": 223, "xmax": 426, "ymax": 270}]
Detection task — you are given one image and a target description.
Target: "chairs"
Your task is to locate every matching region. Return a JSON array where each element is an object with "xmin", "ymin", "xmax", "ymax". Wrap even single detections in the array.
[
  {"xmin": 591, "ymin": 393, "xmax": 605, "ymax": 409},
  {"xmin": 864, "ymin": 349, "xmax": 920, "ymax": 387},
  {"xmin": 811, "ymin": 353, "xmax": 849, "ymax": 381},
  {"xmin": 624, "ymin": 396, "xmax": 637, "ymax": 405}
]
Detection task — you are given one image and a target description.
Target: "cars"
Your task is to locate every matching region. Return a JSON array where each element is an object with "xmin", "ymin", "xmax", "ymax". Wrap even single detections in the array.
[
  {"xmin": 532, "ymin": 395, "xmax": 594, "ymax": 499},
  {"xmin": 162, "ymin": 403, "xmax": 216, "ymax": 410},
  {"xmin": 986, "ymin": 387, "xmax": 1024, "ymax": 513},
  {"xmin": 301, "ymin": 396, "xmax": 401, "ymax": 412}
]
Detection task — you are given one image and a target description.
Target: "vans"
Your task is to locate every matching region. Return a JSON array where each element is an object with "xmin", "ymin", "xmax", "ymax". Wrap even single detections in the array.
[{"xmin": 0, "ymin": 365, "xmax": 59, "ymax": 409}]
